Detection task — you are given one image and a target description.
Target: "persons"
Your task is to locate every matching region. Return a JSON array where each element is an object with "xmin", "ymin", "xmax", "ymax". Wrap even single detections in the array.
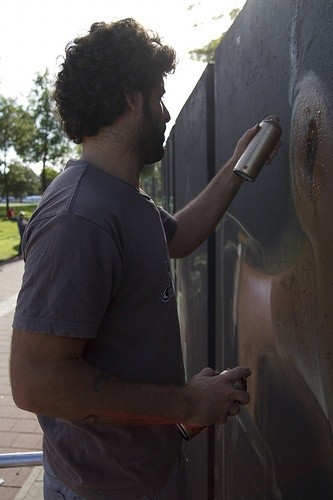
[
  {"xmin": 8, "ymin": 17, "xmax": 282, "ymax": 500},
  {"xmin": 7, "ymin": 206, "xmax": 29, "ymax": 255}
]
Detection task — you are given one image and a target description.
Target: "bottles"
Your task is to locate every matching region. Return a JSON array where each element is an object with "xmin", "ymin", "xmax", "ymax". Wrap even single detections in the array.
[
  {"xmin": 233, "ymin": 115, "xmax": 282, "ymax": 182},
  {"xmin": 176, "ymin": 368, "xmax": 250, "ymax": 441}
]
